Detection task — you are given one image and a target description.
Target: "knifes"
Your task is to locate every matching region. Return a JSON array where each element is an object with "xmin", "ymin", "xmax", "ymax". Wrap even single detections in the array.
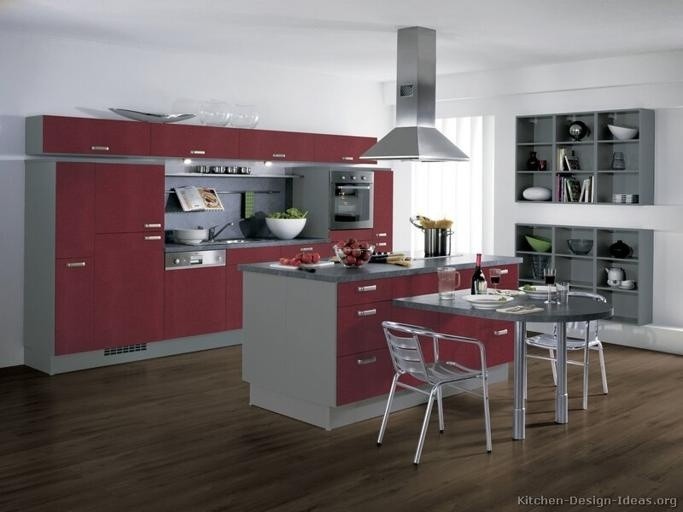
[{"xmin": 268, "ymin": 263, "xmax": 316, "ymax": 273}]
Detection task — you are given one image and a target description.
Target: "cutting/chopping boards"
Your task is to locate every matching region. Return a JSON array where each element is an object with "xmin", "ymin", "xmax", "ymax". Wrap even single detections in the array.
[
  {"xmin": 269, "ymin": 259, "xmax": 334, "ymax": 268},
  {"xmin": 495, "ymin": 306, "xmax": 544, "ymax": 314}
]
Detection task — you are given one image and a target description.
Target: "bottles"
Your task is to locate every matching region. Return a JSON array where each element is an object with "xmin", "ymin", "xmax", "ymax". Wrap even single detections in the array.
[
  {"xmin": 526, "ymin": 151, "xmax": 540, "ymax": 170},
  {"xmin": 471, "ymin": 253, "xmax": 486, "ymax": 294},
  {"xmin": 611, "ymin": 151, "xmax": 625, "ymax": 170}
]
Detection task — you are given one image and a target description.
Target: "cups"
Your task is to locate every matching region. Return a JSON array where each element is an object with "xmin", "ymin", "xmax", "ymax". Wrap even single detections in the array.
[
  {"xmin": 537, "ymin": 160, "xmax": 547, "ymax": 171},
  {"xmin": 621, "ymin": 280, "xmax": 633, "ymax": 287},
  {"xmin": 556, "ymin": 282, "xmax": 568, "ymax": 306}
]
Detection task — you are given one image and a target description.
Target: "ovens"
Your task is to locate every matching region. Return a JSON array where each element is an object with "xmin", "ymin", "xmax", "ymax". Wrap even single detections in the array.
[{"xmin": 329, "ymin": 166, "xmax": 373, "ymax": 230}]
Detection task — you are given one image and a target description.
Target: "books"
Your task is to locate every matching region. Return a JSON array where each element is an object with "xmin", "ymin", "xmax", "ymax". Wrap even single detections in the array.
[
  {"xmin": 557, "ymin": 148, "xmax": 594, "ymax": 203},
  {"xmin": 172, "ymin": 185, "xmax": 225, "ymax": 213}
]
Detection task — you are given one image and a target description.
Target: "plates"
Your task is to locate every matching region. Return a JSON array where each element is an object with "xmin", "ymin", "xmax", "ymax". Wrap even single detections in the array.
[
  {"xmin": 518, "ymin": 285, "xmax": 556, "ymax": 301},
  {"xmin": 462, "ymin": 295, "xmax": 513, "ymax": 311},
  {"xmin": 108, "ymin": 108, "xmax": 195, "ymax": 123},
  {"xmin": 618, "ymin": 286, "xmax": 633, "ymax": 290}
]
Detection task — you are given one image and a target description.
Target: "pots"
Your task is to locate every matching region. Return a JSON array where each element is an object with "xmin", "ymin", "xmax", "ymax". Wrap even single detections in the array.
[{"xmin": 410, "ymin": 215, "xmax": 453, "ymax": 257}]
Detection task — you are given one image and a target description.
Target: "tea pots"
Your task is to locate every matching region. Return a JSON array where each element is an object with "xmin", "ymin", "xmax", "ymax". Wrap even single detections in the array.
[
  {"xmin": 603, "ymin": 266, "xmax": 623, "ymax": 281},
  {"xmin": 436, "ymin": 266, "xmax": 460, "ymax": 300}
]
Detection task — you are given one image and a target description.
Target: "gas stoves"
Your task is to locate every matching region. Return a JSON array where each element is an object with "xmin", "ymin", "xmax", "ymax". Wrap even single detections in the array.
[{"xmin": 411, "ymin": 253, "xmax": 462, "ymax": 260}]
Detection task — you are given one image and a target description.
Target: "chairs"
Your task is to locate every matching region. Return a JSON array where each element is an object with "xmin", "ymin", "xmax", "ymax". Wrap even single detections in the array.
[
  {"xmin": 376, "ymin": 320, "xmax": 492, "ymax": 467},
  {"xmin": 525, "ymin": 291, "xmax": 608, "ymax": 409}
]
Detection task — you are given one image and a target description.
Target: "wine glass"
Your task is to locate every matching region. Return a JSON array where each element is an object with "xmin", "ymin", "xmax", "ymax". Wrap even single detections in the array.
[
  {"xmin": 542, "ymin": 267, "xmax": 556, "ymax": 306},
  {"xmin": 487, "ymin": 268, "xmax": 501, "ymax": 294}
]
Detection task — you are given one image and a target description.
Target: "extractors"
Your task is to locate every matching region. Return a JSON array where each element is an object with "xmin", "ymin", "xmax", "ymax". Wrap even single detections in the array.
[{"xmin": 354, "ymin": 24, "xmax": 469, "ymax": 163}]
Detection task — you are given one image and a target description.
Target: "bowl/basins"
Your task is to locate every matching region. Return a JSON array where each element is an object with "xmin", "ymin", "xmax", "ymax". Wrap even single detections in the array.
[
  {"xmin": 332, "ymin": 243, "xmax": 373, "ymax": 270},
  {"xmin": 524, "ymin": 235, "xmax": 550, "ymax": 252},
  {"xmin": 566, "ymin": 238, "xmax": 593, "ymax": 255},
  {"xmin": 172, "ymin": 227, "xmax": 208, "ymax": 246},
  {"xmin": 196, "ymin": 164, "xmax": 250, "ymax": 174},
  {"xmin": 228, "ymin": 102, "xmax": 259, "ymax": 130},
  {"xmin": 199, "ymin": 101, "xmax": 232, "ymax": 127},
  {"xmin": 264, "ymin": 218, "xmax": 307, "ymax": 240},
  {"xmin": 606, "ymin": 124, "xmax": 637, "ymax": 139}
]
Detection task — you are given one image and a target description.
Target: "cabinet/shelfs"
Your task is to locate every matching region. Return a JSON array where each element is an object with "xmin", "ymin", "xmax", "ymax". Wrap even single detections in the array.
[
  {"xmin": 515, "ymin": 225, "xmax": 640, "ymax": 325},
  {"xmin": 43, "ymin": 115, "xmax": 378, "ymax": 164},
  {"xmin": 234, "ymin": 252, "xmax": 522, "ymax": 407},
  {"xmin": 225, "ymin": 244, "xmax": 329, "ymax": 331},
  {"xmin": 55, "ymin": 162, "xmax": 164, "ymax": 356},
  {"xmin": 293, "ymin": 167, "xmax": 393, "ymax": 258},
  {"xmin": 514, "ymin": 109, "xmax": 640, "ymax": 206}
]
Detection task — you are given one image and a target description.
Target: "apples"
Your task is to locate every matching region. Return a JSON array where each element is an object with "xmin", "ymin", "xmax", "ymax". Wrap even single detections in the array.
[
  {"xmin": 280, "ymin": 251, "xmax": 320, "ymax": 265},
  {"xmin": 338, "ymin": 238, "xmax": 370, "ymax": 265}
]
